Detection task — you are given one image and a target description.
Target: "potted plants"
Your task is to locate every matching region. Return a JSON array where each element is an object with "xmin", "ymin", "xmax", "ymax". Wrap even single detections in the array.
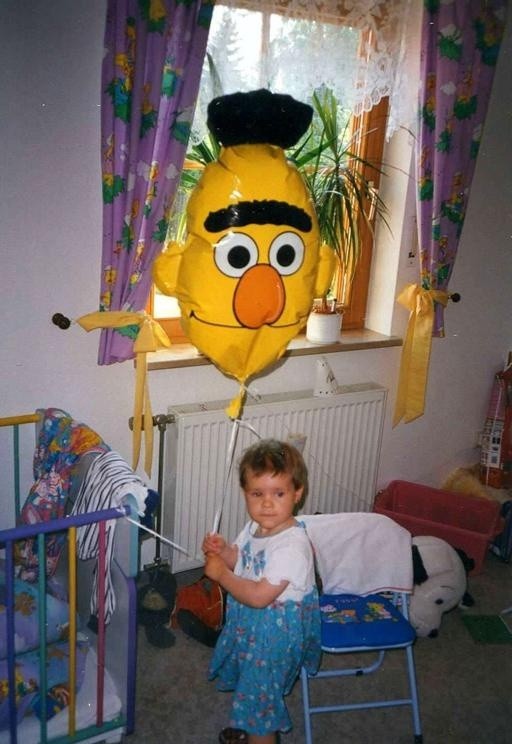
[{"xmin": 287, "ymin": 84, "xmax": 420, "ymax": 345}]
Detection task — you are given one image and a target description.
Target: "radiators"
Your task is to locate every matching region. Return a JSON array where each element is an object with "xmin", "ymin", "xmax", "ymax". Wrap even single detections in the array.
[{"xmin": 162, "ymin": 381, "xmax": 389, "ymax": 575}]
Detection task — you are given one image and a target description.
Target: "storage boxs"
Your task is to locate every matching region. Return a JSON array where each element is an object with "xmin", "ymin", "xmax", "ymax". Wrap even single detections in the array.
[{"xmin": 374, "ymin": 478, "xmax": 505, "ymax": 576}]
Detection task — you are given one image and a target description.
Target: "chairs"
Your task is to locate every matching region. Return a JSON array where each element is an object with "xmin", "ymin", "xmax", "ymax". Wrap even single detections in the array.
[{"xmin": 296, "ymin": 512, "xmax": 423, "ymax": 743}]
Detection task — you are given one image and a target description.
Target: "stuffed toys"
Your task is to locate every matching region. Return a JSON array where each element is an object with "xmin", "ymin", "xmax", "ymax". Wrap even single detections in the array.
[
  {"xmin": 396, "ymin": 533, "xmax": 477, "ymax": 639},
  {"xmin": 136, "ymin": 564, "xmax": 231, "ymax": 651}
]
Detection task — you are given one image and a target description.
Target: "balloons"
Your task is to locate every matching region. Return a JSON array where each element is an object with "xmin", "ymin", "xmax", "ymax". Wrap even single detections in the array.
[{"xmin": 153, "ymin": 86, "xmax": 342, "ymax": 422}]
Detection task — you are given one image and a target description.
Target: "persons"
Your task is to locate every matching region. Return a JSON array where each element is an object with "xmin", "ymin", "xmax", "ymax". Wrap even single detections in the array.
[{"xmin": 199, "ymin": 437, "xmax": 322, "ymax": 744}]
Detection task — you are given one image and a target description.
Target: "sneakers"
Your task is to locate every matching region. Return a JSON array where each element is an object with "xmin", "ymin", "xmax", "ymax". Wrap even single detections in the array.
[{"xmin": 219, "ymin": 727, "xmax": 281, "ymax": 744}]
